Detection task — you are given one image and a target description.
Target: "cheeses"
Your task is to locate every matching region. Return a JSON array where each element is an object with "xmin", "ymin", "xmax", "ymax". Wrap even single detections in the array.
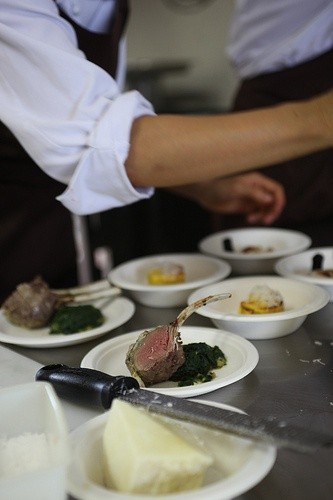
[{"xmin": 103, "ymin": 398, "xmax": 213, "ymax": 495}]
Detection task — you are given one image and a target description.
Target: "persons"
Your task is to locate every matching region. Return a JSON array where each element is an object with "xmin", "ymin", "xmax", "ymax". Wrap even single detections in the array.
[
  {"xmin": 214, "ymin": 0, "xmax": 333, "ymax": 252},
  {"xmin": 0, "ymin": 0, "xmax": 333, "ymax": 310}
]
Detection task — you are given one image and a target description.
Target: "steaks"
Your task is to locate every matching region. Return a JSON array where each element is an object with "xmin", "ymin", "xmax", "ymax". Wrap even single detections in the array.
[
  {"xmin": 126, "ymin": 324, "xmax": 186, "ymax": 388},
  {"xmin": 4, "ymin": 276, "xmax": 56, "ymax": 327}
]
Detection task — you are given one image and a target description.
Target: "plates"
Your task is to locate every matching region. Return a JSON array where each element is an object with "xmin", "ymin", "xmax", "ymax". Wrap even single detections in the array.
[
  {"xmin": 0, "ymin": 295, "xmax": 136, "ymax": 350},
  {"xmin": 79, "ymin": 325, "xmax": 259, "ymax": 399}
]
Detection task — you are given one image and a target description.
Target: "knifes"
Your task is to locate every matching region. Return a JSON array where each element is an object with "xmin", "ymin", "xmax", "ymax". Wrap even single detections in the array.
[{"xmin": 33, "ymin": 361, "xmax": 333, "ymax": 452}]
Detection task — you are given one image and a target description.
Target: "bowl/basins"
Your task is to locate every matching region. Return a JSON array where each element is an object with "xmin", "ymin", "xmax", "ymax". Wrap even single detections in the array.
[
  {"xmin": 60, "ymin": 398, "xmax": 278, "ymax": 499},
  {"xmin": 186, "ymin": 275, "xmax": 329, "ymax": 341},
  {"xmin": 108, "ymin": 254, "xmax": 231, "ymax": 308},
  {"xmin": 199, "ymin": 228, "xmax": 312, "ymax": 274},
  {"xmin": 273, "ymin": 245, "xmax": 333, "ymax": 301}
]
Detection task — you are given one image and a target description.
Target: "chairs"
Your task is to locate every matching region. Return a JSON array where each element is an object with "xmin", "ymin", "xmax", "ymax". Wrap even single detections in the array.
[{"xmin": 72, "ymin": 189, "xmax": 213, "ymax": 286}]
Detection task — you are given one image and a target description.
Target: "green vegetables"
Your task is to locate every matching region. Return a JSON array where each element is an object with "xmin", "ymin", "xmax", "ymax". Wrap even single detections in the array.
[
  {"xmin": 49, "ymin": 305, "xmax": 104, "ymax": 334},
  {"xmin": 169, "ymin": 342, "xmax": 227, "ymax": 388}
]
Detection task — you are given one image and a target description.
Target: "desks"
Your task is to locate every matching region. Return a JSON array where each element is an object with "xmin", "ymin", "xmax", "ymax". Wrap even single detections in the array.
[{"xmin": 0, "ymin": 273, "xmax": 332, "ymax": 500}]
